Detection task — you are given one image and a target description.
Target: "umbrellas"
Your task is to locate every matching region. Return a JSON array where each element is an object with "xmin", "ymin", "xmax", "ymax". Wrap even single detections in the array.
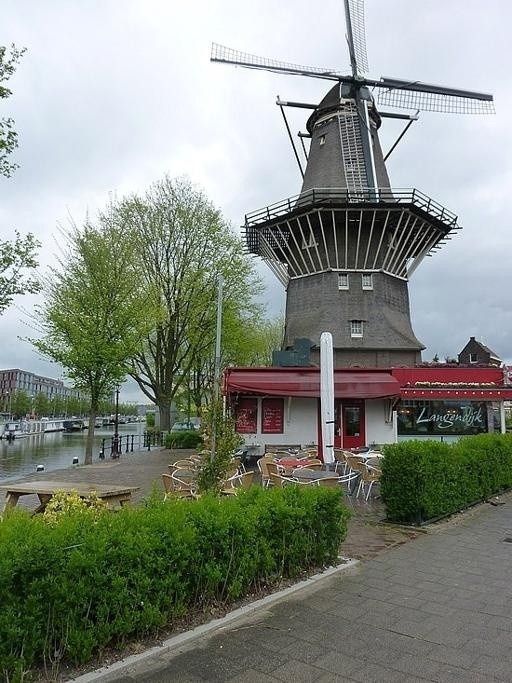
[{"xmin": 319, "ymin": 331, "xmax": 335, "ymax": 471}]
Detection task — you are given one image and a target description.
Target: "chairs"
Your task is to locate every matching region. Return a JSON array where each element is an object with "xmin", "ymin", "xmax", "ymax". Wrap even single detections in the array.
[{"xmin": 160, "ymin": 442, "xmax": 389, "ymax": 505}]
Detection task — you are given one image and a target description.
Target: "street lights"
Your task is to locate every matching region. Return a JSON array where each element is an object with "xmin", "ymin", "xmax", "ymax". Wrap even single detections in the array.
[
  {"xmin": 63, "ymin": 398, "xmax": 66, "ymax": 413},
  {"xmin": 5, "ymin": 390, "xmax": 13, "ymax": 421},
  {"xmin": 51, "ymin": 396, "xmax": 56, "ymax": 415},
  {"xmin": 30, "ymin": 394, "xmax": 34, "ymax": 415},
  {"xmin": 109, "ymin": 380, "xmax": 123, "ymax": 457},
  {"xmin": 77, "ymin": 399, "xmax": 81, "ymax": 415}
]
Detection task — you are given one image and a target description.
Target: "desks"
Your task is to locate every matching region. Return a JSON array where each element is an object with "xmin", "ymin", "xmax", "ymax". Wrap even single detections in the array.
[{"xmin": 0, "ymin": 478, "xmax": 141, "ymax": 514}]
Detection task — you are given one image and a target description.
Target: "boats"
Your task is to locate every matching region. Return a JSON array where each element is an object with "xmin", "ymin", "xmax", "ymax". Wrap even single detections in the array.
[
  {"xmin": 0, "ymin": 421, "xmax": 33, "ymax": 439},
  {"xmin": 62, "ymin": 412, "xmax": 146, "ymax": 434}
]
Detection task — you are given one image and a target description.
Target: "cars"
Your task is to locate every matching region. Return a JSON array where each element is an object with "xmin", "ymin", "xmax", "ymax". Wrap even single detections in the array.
[
  {"xmin": 16, "ymin": 414, "xmax": 61, "ymax": 421},
  {"xmin": 182, "ymin": 415, "xmax": 203, "ymax": 430},
  {"xmin": 169, "ymin": 420, "xmax": 196, "ymax": 433}
]
